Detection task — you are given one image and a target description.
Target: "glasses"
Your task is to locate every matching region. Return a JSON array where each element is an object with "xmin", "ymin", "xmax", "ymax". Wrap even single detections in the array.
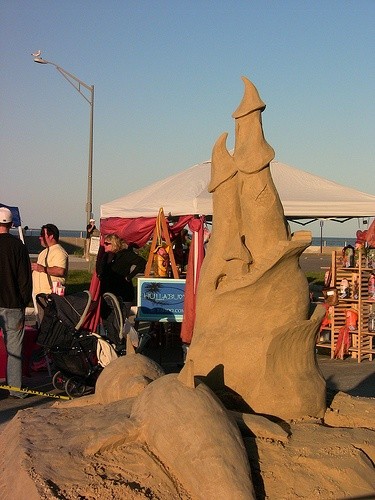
[{"xmin": 104, "ymin": 242, "xmax": 111, "ymax": 246}]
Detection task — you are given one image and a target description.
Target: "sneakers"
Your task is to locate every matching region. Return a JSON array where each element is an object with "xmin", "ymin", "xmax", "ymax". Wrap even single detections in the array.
[{"xmin": 8, "ymin": 387, "xmax": 28, "ymax": 400}]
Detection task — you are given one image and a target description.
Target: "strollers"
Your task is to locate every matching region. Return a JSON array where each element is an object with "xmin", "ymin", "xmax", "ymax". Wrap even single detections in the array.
[{"xmin": 36, "ymin": 293, "xmax": 139, "ymax": 399}]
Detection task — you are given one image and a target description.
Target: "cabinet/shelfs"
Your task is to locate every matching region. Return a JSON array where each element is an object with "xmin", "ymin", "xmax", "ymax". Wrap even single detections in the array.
[{"xmin": 315, "ymin": 250, "xmax": 375, "ymax": 363}]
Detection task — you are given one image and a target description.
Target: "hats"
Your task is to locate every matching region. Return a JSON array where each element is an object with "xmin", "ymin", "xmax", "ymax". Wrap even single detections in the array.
[
  {"xmin": 90, "ymin": 219, "xmax": 95, "ymax": 222},
  {"xmin": 0, "ymin": 207, "xmax": 13, "ymax": 223}
]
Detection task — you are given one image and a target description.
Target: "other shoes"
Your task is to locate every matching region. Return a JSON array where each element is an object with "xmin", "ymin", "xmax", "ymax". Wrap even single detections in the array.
[{"xmin": 86, "ymin": 259, "xmax": 90, "ymax": 261}]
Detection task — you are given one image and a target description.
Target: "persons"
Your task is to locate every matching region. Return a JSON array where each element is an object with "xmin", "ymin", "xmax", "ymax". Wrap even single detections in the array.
[
  {"xmin": 96, "ymin": 233, "xmax": 147, "ymax": 353},
  {"xmin": 85, "ymin": 219, "xmax": 96, "ymax": 261},
  {"xmin": 0, "ymin": 207, "xmax": 33, "ymax": 398},
  {"xmin": 18, "ymin": 226, "xmax": 28, "ymax": 244},
  {"xmin": 31, "ymin": 224, "xmax": 69, "ymax": 383}
]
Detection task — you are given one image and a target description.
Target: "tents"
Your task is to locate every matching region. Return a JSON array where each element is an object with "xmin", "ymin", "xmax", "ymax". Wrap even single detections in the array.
[
  {"xmin": 0, "ymin": 203, "xmax": 26, "ymax": 245},
  {"xmin": 82, "ymin": 150, "xmax": 375, "ymax": 355}
]
